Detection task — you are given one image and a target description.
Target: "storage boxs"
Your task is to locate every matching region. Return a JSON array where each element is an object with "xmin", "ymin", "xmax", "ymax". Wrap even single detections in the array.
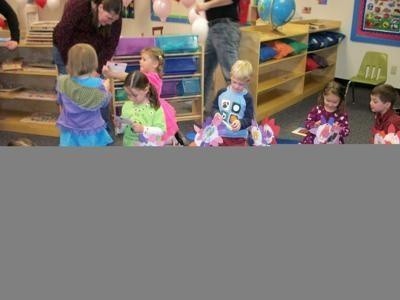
[{"xmin": 110, "ymin": 34, "xmax": 200, "ymax": 103}]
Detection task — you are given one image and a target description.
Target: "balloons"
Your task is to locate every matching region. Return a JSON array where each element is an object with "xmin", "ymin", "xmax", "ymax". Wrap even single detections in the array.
[
  {"xmin": 181, "ymin": 0, "xmax": 210, "ymax": 46},
  {"xmin": 152, "ymin": 0, "xmax": 171, "ymax": 25},
  {"xmin": 36, "ymin": 0, "xmax": 48, "ymax": 8}
]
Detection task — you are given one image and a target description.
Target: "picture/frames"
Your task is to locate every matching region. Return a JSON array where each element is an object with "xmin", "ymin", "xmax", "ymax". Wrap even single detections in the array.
[{"xmin": 347, "ymin": 0, "xmax": 400, "ymax": 47}]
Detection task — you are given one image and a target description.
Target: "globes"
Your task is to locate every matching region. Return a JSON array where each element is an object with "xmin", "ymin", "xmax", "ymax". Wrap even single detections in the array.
[{"xmin": 258, "ymin": 0, "xmax": 296, "ymax": 36}]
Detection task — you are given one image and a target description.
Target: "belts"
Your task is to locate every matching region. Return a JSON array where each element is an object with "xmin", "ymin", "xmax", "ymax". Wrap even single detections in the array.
[{"xmin": 207, "ymin": 18, "xmax": 236, "ymax": 26}]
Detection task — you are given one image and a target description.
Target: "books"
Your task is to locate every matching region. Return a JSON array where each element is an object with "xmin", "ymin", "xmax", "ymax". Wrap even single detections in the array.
[
  {"xmin": 19, "ymin": 114, "xmax": 60, "ymax": 125},
  {"xmin": 27, "ymin": 87, "xmax": 57, "ymax": 98},
  {"xmin": 25, "ymin": 21, "xmax": 60, "ymax": 46},
  {"xmin": 0, "ymin": 84, "xmax": 24, "ymax": 93},
  {"xmin": 29, "ymin": 110, "xmax": 58, "ymax": 123},
  {"xmin": 2, "ymin": 55, "xmax": 26, "ymax": 72},
  {"xmin": 28, "ymin": 60, "xmax": 57, "ymax": 68}
]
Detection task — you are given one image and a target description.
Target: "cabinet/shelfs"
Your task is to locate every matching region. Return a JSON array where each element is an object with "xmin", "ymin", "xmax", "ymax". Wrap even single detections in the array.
[
  {"xmin": 236, "ymin": 18, "xmax": 341, "ymax": 124},
  {"xmin": 0, "ymin": 29, "xmax": 62, "ymax": 140},
  {"xmin": 105, "ymin": 34, "xmax": 205, "ymax": 136}
]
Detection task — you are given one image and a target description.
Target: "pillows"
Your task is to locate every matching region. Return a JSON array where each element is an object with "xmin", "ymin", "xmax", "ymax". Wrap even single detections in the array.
[{"xmin": 260, "ymin": 31, "xmax": 347, "ymax": 72}]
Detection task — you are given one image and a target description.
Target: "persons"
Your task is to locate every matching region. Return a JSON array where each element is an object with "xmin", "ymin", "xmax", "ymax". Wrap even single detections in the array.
[
  {"xmin": 112, "ymin": 69, "xmax": 168, "ymax": 147},
  {"xmin": 208, "ymin": 59, "xmax": 256, "ymax": 146},
  {"xmin": 0, "ymin": 0, "xmax": 20, "ymax": 50},
  {"xmin": 138, "ymin": 45, "xmax": 188, "ymax": 146},
  {"xmin": 51, "ymin": 0, "xmax": 124, "ymax": 75},
  {"xmin": 301, "ymin": 81, "xmax": 350, "ymax": 144},
  {"xmin": 367, "ymin": 84, "xmax": 400, "ymax": 145},
  {"xmin": 195, "ymin": 0, "xmax": 244, "ymax": 114},
  {"xmin": 52, "ymin": 43, "xmax": 116, "ymax": 147}
]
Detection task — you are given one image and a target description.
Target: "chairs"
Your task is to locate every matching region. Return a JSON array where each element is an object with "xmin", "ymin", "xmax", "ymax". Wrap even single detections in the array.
[{"xmin": 342, "ymin": 51, "xmax": 389, "ymax": 115}]
